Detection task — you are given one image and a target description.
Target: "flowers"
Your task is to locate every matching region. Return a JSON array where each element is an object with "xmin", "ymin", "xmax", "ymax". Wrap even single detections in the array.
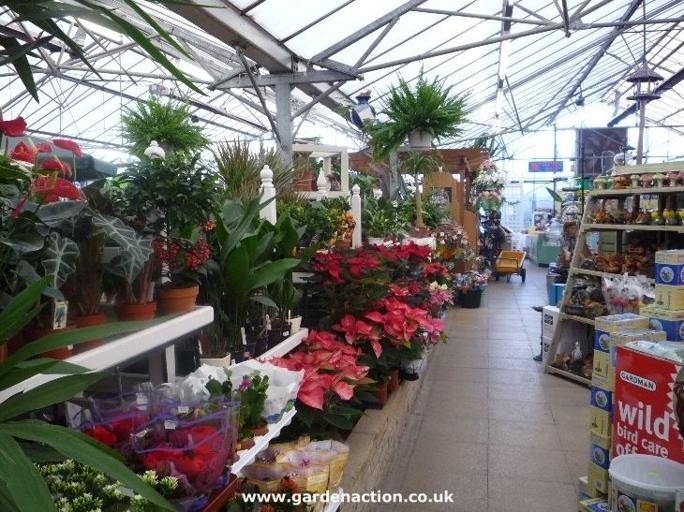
[{"xmin": 1, "ymin": 109, "xmax": 504, "ymax": 512}]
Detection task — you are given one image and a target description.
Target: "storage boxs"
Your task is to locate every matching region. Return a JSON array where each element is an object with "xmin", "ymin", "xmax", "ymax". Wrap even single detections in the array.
[
  {"xmin": 542, "ymin": 336, "xmax": 552, "ymax": 362},
  {"xmin": 575, "ymin": 249, "xmax": 684, "ymax": 511},
  {"xmin": 541, "ymin": 304, "xmax": 561, "ymax": 339}
]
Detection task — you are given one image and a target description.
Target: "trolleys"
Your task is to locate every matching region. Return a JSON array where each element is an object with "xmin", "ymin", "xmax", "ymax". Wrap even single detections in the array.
[{"xmin": 492, "ymin": 249, "xmax": 529, "ymax": 285}]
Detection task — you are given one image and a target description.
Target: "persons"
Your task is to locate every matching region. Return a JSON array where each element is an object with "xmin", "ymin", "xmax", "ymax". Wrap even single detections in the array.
[{"xmin": 557, "ymin": 219, "xmax": 592, "ymax": 264}]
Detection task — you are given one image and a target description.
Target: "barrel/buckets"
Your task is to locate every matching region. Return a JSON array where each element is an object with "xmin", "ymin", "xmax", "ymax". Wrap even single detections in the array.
[{"xmin": 609, "ymin": 453, "xmax": 684, "ymax": 512}]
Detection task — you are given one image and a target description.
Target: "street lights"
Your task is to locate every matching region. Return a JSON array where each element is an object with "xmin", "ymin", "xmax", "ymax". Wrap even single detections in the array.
[{"xmin": 627, "ymin": 59, "xmax": 664, "ymax": 165}]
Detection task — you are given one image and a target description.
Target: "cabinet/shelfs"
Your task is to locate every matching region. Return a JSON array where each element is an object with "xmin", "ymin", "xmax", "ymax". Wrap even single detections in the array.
[{"xmin": 538, "ymin": 157, "xmax": 684, "ymax": 390}]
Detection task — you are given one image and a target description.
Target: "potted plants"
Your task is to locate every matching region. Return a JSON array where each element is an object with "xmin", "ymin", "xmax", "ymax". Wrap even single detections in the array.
[{"xmin": 357, "ymin": 64, "xmax": 475, "ymax": 166}]
